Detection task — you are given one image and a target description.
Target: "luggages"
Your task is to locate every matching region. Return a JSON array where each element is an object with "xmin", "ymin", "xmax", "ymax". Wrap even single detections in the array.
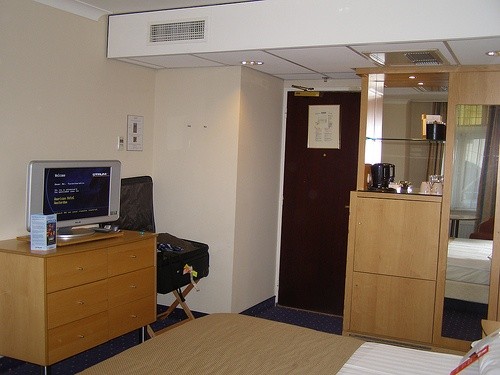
[{"xmin": 103, "ymin": 175, "xmax": 209, "ymax": 294}]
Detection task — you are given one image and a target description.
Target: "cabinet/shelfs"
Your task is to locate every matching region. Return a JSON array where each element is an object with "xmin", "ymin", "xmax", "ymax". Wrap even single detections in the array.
[
  {"xmin": 0, "ymin": 228, "xmax": 160, "ymax": 374},
  {"xmin": 342, "ymin": 65, "xmax": 458, "ymax": 344}
]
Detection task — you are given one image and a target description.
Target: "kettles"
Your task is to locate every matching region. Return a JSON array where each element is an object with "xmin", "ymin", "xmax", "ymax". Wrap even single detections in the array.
[
  {"xmin": 383, "ymin": 163, "xmax": 396, "ymax": 188},
  {"xmin": 370, "ymin": 162, "xmax": 390, "ymax": 188}
]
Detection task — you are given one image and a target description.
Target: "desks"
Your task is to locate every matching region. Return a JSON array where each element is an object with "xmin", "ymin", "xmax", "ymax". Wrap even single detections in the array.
[{"xmin": 79, "ymin": 312, "xmax": 500, "ymax": 375}]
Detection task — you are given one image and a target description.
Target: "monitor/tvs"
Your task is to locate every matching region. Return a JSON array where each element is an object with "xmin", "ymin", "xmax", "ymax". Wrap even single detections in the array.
[{"xmin": 25, "ymin": 160, "xmax": 121, "ymax": 229}]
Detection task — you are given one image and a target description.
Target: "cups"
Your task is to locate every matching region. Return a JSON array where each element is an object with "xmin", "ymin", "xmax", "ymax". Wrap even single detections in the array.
[{"xmin": 423, "ymin": 175, "xmax": 443, "ymax": 195}]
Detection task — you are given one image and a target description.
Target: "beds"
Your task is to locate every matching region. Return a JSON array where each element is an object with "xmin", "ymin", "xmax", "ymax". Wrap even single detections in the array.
[{"xmin": 444, "ymin": 236, "xmax": 494, "ymax": 305}]
